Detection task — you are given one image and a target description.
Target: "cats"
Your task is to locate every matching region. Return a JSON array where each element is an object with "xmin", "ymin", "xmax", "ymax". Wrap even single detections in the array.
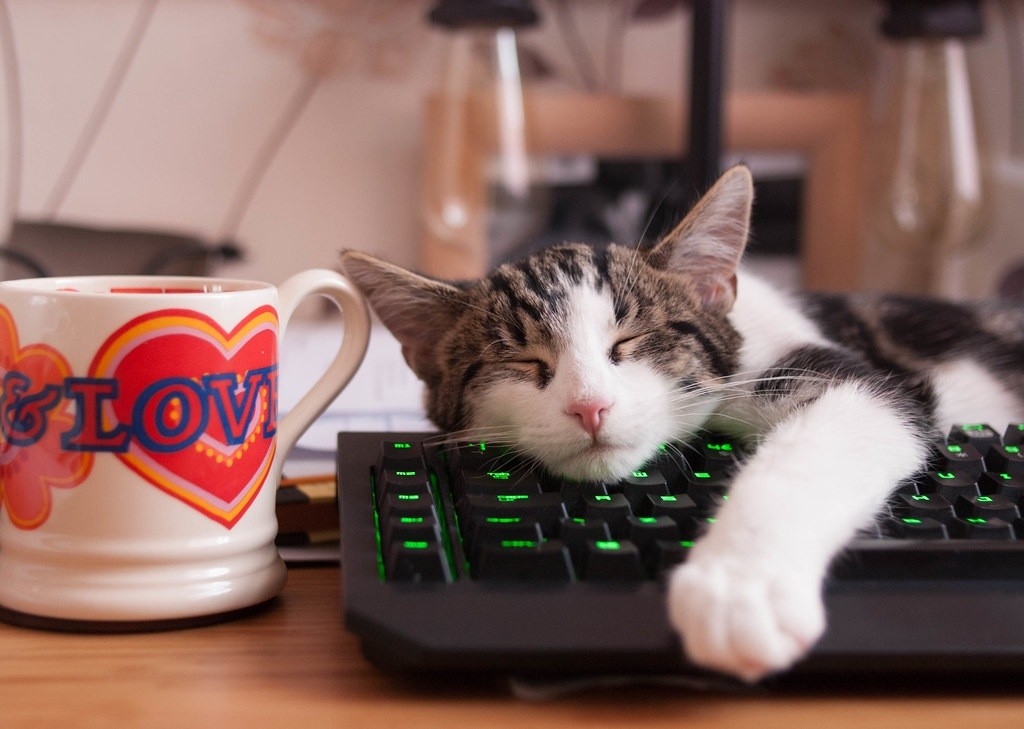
[{"xmin": 337, "ymin": 159, "xmax": 1024, "ymax": 687}]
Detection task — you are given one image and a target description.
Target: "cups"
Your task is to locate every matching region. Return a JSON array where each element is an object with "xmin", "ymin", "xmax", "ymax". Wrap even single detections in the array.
[{"xmin": 0, "ymin": 269, "xmax": 374, "ymax": 636}]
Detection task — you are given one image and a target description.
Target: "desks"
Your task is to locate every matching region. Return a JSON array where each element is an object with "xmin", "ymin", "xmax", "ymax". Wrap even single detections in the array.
[{"xmin": 0, "ymin": 562, "xmax": 1024, "ymax": 729}]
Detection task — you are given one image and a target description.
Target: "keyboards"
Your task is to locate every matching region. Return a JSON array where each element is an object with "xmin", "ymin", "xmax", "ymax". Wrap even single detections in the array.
[{"xmin": 337, "ymin": 419, "xmax": 1024, "ymax": 703}]
